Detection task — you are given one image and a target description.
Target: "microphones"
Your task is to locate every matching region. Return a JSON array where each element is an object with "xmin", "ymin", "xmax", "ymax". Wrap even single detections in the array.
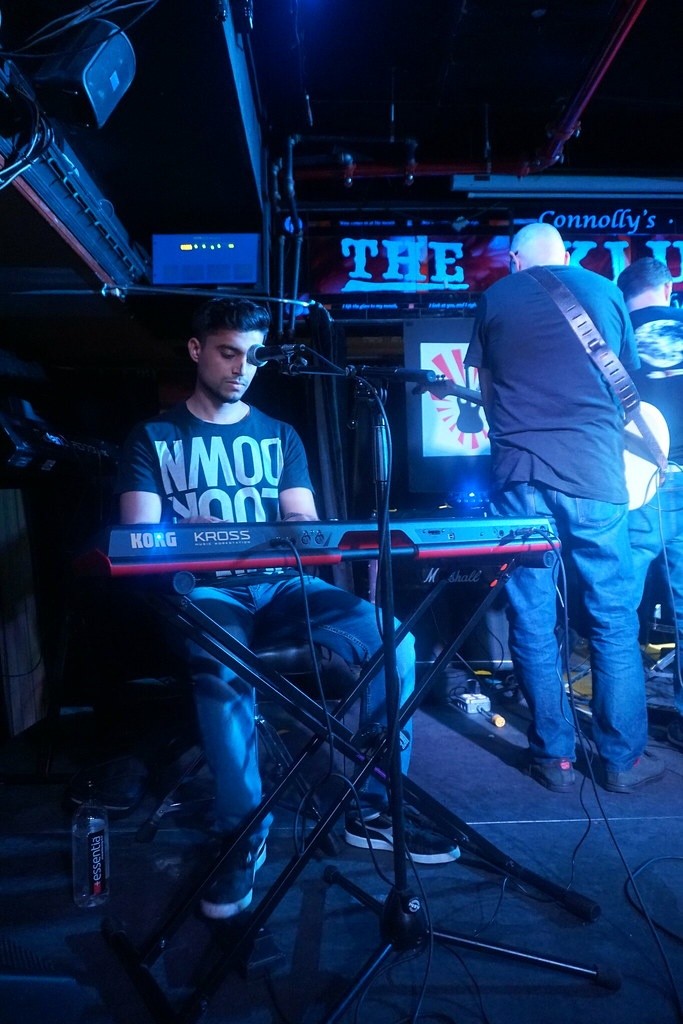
[{"xmin": 247, "ymin": 344, "xmax": 306, "ymax": 367}]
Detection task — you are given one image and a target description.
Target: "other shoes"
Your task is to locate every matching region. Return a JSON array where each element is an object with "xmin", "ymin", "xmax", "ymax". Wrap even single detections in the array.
[
  {"xmin": 530, "ymin": 760, "xmax": 576, "ymax": 792},
  {"xmin": 666, "ymin": 719, "xmax": 683, "ymax": 748},
  {"xmin": 605, "ymin": 750, "xmax": 665, "ymax": 793}
]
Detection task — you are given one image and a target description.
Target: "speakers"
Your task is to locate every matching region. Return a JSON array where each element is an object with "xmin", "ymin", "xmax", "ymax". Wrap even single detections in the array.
[{"xmin": 30, "ymin": 18, "xmax": 136, "ymax": 130}]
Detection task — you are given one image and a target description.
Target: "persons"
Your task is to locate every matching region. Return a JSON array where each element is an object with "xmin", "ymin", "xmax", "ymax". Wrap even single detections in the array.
[
  {"xmin": 120, "ymin": 299, "xmax": 463, "ymax": 919},
  {"xmin": 617, "ymin": 258, "xmax": 683, "ymax": 746},
  {"xmin": 462, "ymin": 223, "xmax": 666, "ymax": 795}
]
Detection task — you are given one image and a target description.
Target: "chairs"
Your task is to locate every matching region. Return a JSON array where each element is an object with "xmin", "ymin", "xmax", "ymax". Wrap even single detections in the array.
[{"xmin": 122, "ymin": 563, "xmax": 361, "ymax": 858}]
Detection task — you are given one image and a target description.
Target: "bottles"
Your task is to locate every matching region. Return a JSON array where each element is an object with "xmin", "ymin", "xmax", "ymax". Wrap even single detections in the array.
[{"xmin": 70, "ymin": 776, "xmax": 113, "ymax": 907}]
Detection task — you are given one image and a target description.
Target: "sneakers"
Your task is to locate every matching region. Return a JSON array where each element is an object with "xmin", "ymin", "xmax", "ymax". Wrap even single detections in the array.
[
  {"xmin": 343, "ymin": 810, "xmax": 461, "ymax": 863},
  {"xmin": 198, "ymin": 837, "xmax": 268, "ymax": 920}
]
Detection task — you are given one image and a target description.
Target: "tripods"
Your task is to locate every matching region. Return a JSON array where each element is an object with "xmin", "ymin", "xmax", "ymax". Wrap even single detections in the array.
[{"xmin": 279, "ymin": 356, "xmax": 626, "ymax": 1024}]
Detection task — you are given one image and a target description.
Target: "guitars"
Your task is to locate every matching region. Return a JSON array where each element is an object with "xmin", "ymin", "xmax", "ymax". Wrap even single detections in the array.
[{"xmin": 411, "ymin": 374, "xmax": 670, "ymax": 511}]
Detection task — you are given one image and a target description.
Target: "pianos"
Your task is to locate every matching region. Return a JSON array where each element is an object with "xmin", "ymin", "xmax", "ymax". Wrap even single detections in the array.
[{"xmin": 71, "ymin": 514, "xmax": 563, "ymax": 585}]
